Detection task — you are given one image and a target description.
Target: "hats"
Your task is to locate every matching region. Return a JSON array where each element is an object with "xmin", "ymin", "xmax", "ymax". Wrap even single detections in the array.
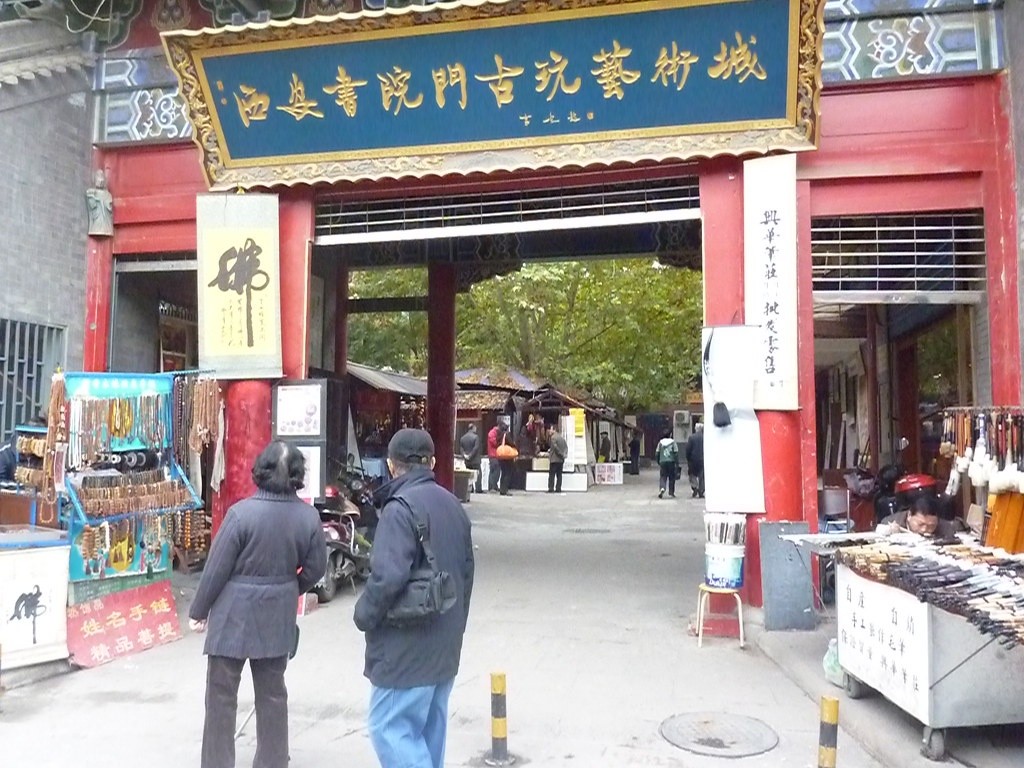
[
  {"xmin": 386, "ymin": 428, "xmax": 435, "ymax": 464},
  {"xmin": 599, "ymin": 431, "xmax": 608, "ymax": 434}
]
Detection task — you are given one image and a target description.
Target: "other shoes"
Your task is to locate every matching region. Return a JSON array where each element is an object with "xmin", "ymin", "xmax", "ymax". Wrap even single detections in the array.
[
  {"xmin": 657, "ymin": 487, "xmax": 666, "ymax": 498},
  {"xmin": 698, "ymin": 492, "xmax": 704, "ymax": 498},
  {"xmin": 668, "ymin": 493, "xmax": 676, "ymax": 498},
  {"xmin": 499, "ymin": 491, "xmax": 513, "ymax": 496},
  {"xmin": 692, "ymin": 489, "xmax": 698, "ymax": 497}
]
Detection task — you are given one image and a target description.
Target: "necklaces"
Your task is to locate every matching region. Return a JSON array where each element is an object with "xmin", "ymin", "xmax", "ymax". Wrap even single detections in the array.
[{"xmin": 40, "ymin": 373, "xmax": 220, "ymax": 524}]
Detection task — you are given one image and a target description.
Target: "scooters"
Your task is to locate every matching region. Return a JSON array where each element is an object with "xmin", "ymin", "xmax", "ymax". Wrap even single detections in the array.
[
  {"xmin": 857, "ymin": 437, "xmax": 938, "ymax": 523},
  {"xmin": 305, "ymin": 453, "xmax": 383, "ymax": 603}
]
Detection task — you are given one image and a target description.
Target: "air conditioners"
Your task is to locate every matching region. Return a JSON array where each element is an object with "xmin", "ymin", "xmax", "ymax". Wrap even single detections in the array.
[{"xmin": 673, "ymin": 410, "xmax": 691, "ymax": 424}]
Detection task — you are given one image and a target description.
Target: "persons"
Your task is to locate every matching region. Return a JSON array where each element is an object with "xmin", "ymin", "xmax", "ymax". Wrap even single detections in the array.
[
  {"xmin": 880, "ymin": 496, "xmax": 957, "ymax": 541},
  {"xmin": 685, "ymin": 422, "xmax": 704, "ymax": 498},
  {"xmin": 353, "ymin": 428, "xmax": 474, "ymax": 768},
  {"xmin": 655, "ymin": 428, "xmax": 679, "ymax": 498},
  {"xmin": 600, "ymin": 431, "xmax": 611, "ymax": 462},
  {"xmin": 546, "ymin": 424, "xmax": 568, "ymax": 492},
  {"xmin": 459, "ymin": 421, "xmax": 539, "ymax": 496},
  {"xmin": 188, "ymin": 440, "xmax": 327, "ymax": 768},
  {"xmin": 628, "ymin": 432, "xmax": 640, "ymax": 475}
]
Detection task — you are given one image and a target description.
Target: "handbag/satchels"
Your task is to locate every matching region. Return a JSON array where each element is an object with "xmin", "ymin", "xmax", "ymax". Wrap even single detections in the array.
[
  {"xmin": 495, "ymin": 433, "xmax": 518, "ymax": 460},
  {"xmin": 674, "ymin": 463, "xmax": 682, "ymax": 480},
  {"xmin": 597, "ymin": 451, "xmax": 605, "ymax": 463},
  {"xmin": 377, "ymin": 494, "xmax": 457, "ymax": 627}
]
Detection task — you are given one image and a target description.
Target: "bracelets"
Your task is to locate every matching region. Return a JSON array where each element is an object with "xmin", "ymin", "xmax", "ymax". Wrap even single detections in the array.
[
  {"xmin": 14, "ymin": 435, "xmax": 47, "ymax": 486},
  {"xmin": 82, "ymin": 452, "xmax": 205, "ymax": 580}
]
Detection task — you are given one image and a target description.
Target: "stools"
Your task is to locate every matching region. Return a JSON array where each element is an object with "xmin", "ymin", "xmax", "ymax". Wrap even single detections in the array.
[{"xmin": 694, "ymin": 584, "xmax": 746, "ymax": 649}]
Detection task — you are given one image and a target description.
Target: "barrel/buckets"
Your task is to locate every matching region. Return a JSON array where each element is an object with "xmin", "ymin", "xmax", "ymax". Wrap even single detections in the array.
[{"xmin": 703, "ymin": 540, "xmax": 746, "ymax": 589}]
